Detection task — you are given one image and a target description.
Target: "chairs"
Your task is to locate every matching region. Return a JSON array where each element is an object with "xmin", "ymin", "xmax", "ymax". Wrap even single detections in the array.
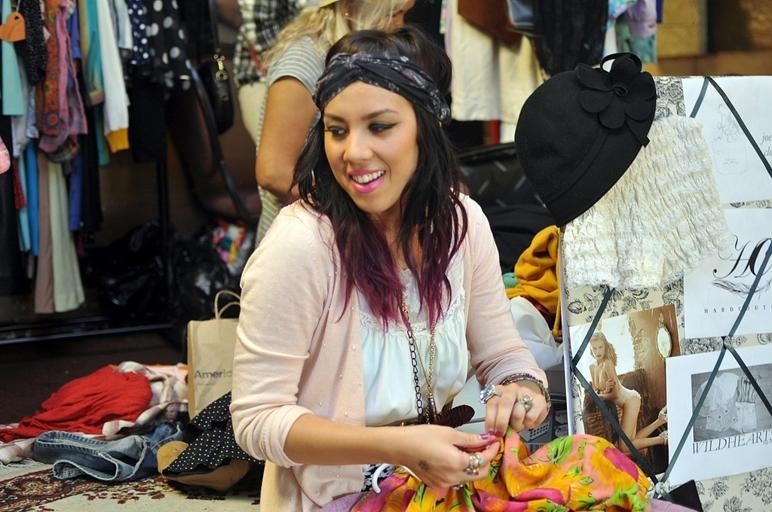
[{"xmin": 166, "ymin": 55, "xmax": 265, "ymax": 226}]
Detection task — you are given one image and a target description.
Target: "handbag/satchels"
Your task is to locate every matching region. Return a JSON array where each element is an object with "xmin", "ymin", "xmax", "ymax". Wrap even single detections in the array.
[
  {"xmin": 197, "ymin": 56, "xmax": 234, "ymax": 134},
  {"xmin": 186, "ymin": 318, "xmax": 238, "ymax": 422},
  {"xmin": 93, "ymin": 222, "xmax": 254, "ymax": 334}
]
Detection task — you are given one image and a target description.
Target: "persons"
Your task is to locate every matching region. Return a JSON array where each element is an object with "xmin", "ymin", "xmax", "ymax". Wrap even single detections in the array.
[
  {"xmin": 226, "ymin": 28, "xmax": 552, "ymax": 512},
  {"xmin": 215, "ymin": 0, "xmax": 325, "ymax": 144},
  {"xmin": 586, "ymin": 332, "xmax": 670, "ymax": 449},
  {"xmin": 251, "ymin": 1, "xmax": 422, "ymax": 251}
]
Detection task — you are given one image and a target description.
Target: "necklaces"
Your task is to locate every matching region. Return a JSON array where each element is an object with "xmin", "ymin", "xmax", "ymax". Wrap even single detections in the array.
[{"xmin": 393, "ymin": 282, "xmax": 442, "ymax": 425}]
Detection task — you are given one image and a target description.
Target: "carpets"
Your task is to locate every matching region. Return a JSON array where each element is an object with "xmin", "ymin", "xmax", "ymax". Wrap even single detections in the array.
[{"xmin": 1, "ymin": 445, "xmax": 260, "ymax": 511}]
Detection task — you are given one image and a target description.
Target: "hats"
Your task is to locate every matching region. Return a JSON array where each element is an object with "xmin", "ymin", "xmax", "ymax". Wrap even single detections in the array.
[{"xmin": 515, "ymin": 53, "xmax": 655, "ymax": 228}]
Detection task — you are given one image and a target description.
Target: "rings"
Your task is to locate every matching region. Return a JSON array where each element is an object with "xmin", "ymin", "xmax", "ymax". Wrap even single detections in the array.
[
  {"xmin": 479, "ymin": 382, "xmax": 503, "ymax": 402},
  {"xmin": 517, "ymin": 392, "xmax": 534, "ymax": 411},
  {"xmin": 465, "ymin": 451, "xmax": 484, "ymax": 476}
]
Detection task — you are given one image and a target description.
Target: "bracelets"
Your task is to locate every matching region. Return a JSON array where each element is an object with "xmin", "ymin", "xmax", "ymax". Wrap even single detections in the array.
[{"xmin": 498, "ymin": 372, "xmax": 552, "ymax": 410}]
